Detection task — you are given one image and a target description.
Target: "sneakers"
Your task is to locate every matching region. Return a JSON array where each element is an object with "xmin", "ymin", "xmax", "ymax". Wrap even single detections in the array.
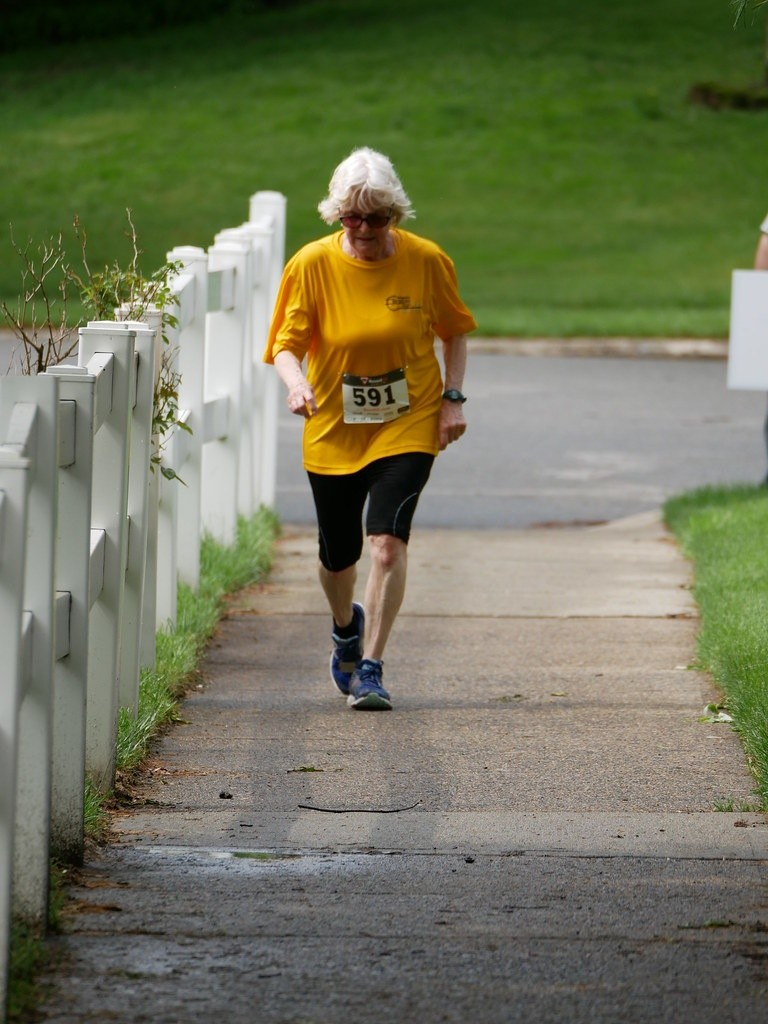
[
  {"xmin": 330, "ymin": 602, "xmax": 367, "ymax": 697},
  {"xmin": 347, "ymin": 659, "xmax": 393, "ymax": 710}
]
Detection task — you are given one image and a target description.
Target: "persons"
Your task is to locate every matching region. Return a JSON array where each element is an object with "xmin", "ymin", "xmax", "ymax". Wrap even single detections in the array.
[{"xmin": 263, "ymin": 146, "xmax": 471, "ymax": 709}]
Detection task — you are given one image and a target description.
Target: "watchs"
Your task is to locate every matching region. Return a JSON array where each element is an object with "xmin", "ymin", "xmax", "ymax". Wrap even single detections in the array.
[{"xmin": 442, "ymin": 389, "xmax": 468, "ymax": 402}]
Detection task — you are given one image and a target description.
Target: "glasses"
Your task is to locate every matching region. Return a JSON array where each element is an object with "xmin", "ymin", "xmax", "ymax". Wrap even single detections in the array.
[{"xmin": 340, "ymin": 208, "xmax": 393, "ymax": 229}]
